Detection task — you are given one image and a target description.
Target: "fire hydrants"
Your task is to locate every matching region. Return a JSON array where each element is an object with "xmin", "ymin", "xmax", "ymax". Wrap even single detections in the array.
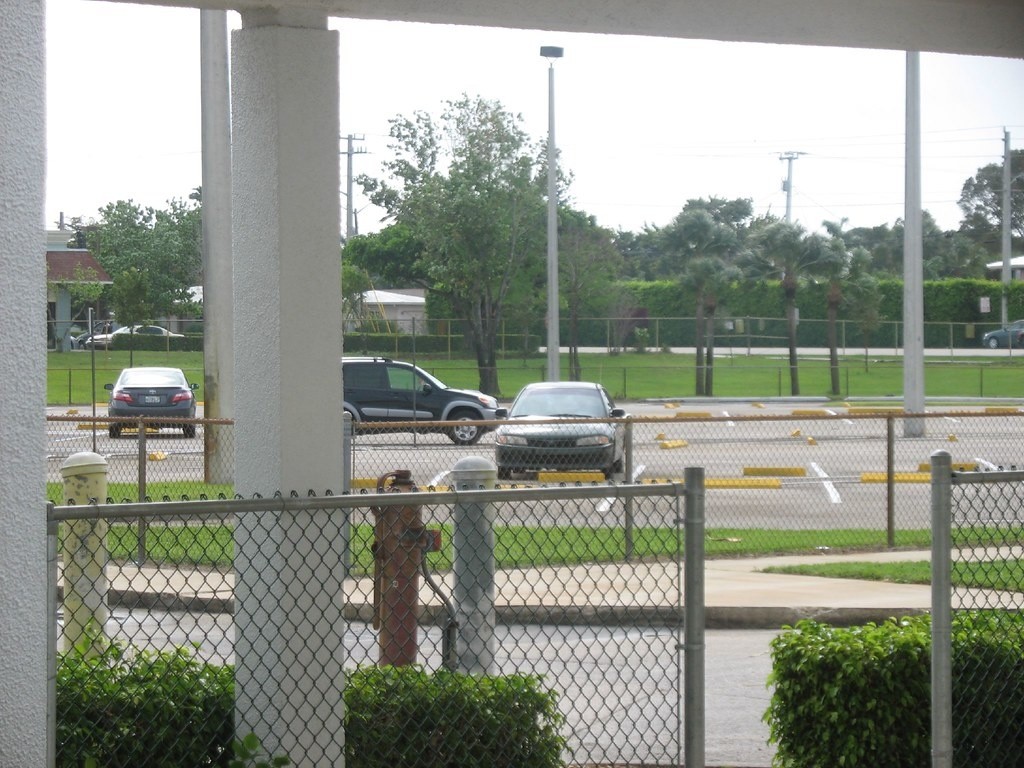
[{"xmin": 369, "ymin": 469, "xmax": 441, "ymax": 669}]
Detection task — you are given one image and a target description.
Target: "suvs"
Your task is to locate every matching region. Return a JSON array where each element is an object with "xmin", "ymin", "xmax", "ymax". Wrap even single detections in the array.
[{"xmin": 342, "ymin": 357, "xmax": 501, "ymax": 445}]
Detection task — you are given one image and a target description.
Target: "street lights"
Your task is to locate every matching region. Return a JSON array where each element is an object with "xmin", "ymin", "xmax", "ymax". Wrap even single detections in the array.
[{"xmin": 540, "ymin": 44, "xmax": 562, "ymax": 383}]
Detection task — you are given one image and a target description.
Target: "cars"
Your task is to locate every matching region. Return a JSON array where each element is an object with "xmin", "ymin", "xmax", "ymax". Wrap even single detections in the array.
[
  {"xmin": 104, "ymin": 367, "xmax": 200, "ymax": 438},
  {"xmin": 983, "ymin": 319, "xmax": 1024, "ymax": 349},
  {"xmin": 494, "ymin": 382, "xmax": 630, "ymax": 480},
  {"xmin": 76, "ymin": 320, "xmax": 184, "ymax": 351}
]
